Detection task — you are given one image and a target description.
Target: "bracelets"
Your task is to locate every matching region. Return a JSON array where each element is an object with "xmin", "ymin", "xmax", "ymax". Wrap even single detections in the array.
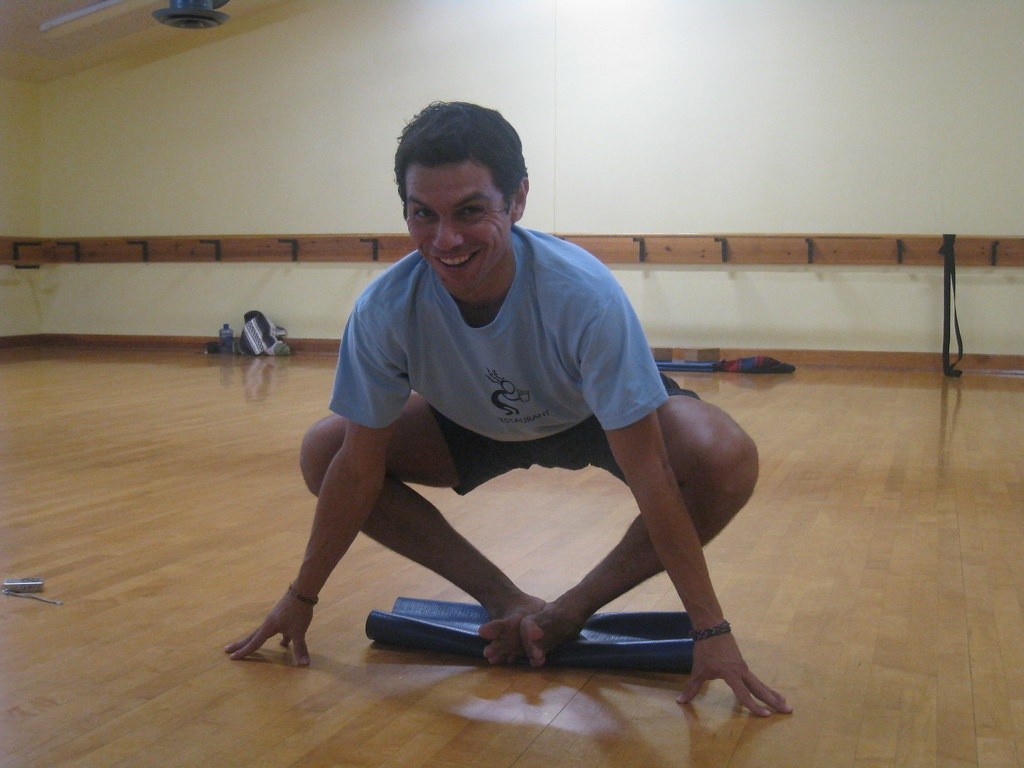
[
  {"xmin": 288, "ymin": 587, "xmax": 319, "ymax": 605},
  {"xmin": 691, "ymin": 620, "xmax": 730, "ymax": 641}
]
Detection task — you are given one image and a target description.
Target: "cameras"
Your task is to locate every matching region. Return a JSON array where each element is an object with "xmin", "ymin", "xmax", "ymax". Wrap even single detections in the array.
[{"xmin": 3, "ymin": 576, "xmax": 45, "ymax": 594}]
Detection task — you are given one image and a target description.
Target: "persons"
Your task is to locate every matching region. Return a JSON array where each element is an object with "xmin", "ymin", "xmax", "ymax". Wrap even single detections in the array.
[{"xmin": 224, "ymin": 101, "xmax": 794, "ymax": 718}]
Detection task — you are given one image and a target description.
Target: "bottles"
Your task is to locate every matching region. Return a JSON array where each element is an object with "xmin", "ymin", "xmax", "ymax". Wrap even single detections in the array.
[{"xmin": 219, "ymin": 324, "xmax": 233, "ymax": 353}]
